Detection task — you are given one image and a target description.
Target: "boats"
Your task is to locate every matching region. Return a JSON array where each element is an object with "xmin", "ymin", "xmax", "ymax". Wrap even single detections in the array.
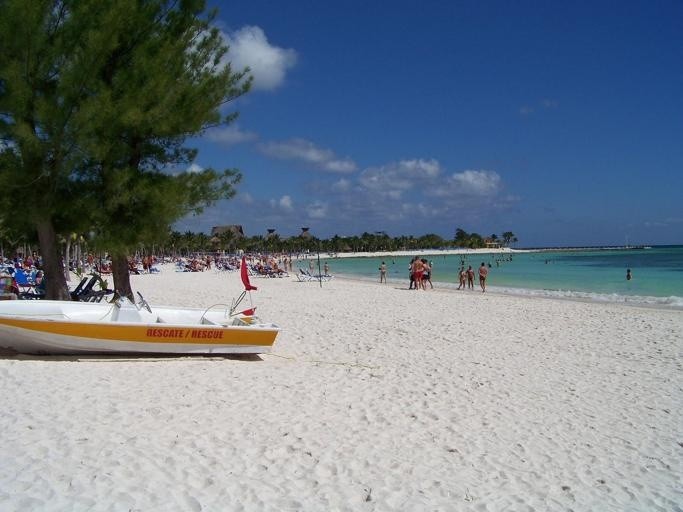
[{"xmin": 0, "ymin": 300, "xmax": 282, "ymax": 354}]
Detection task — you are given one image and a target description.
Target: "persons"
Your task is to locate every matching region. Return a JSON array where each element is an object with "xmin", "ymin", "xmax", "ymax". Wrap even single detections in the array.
[
  {"xmin": 456, "ymin": 252, "xmax": 513, "ymax": 293},
  {"xmin": 378, "ymin": 258, "xmax": 395, "ymax": 284},
  {"xmin": 544, "ymin": 259, "xmax": 552, "ymax": 264},
  {"xmin": 626, "ymin": 268, "xmax": 632, "ymax": 280},
  {"xmin": 323, "ymin": 261, "xmax": 329, "ymax": 274},
  {"xmin": 408, "ymin": 255, "xmax": 433, "ymax": 291},
  {"xmin": 309, "ymin": 259, "xmax": 315, "ymax": 274},
  {"xmin": 0, "ymin": 250, "xmax": 294, "ymax": 293}
]
